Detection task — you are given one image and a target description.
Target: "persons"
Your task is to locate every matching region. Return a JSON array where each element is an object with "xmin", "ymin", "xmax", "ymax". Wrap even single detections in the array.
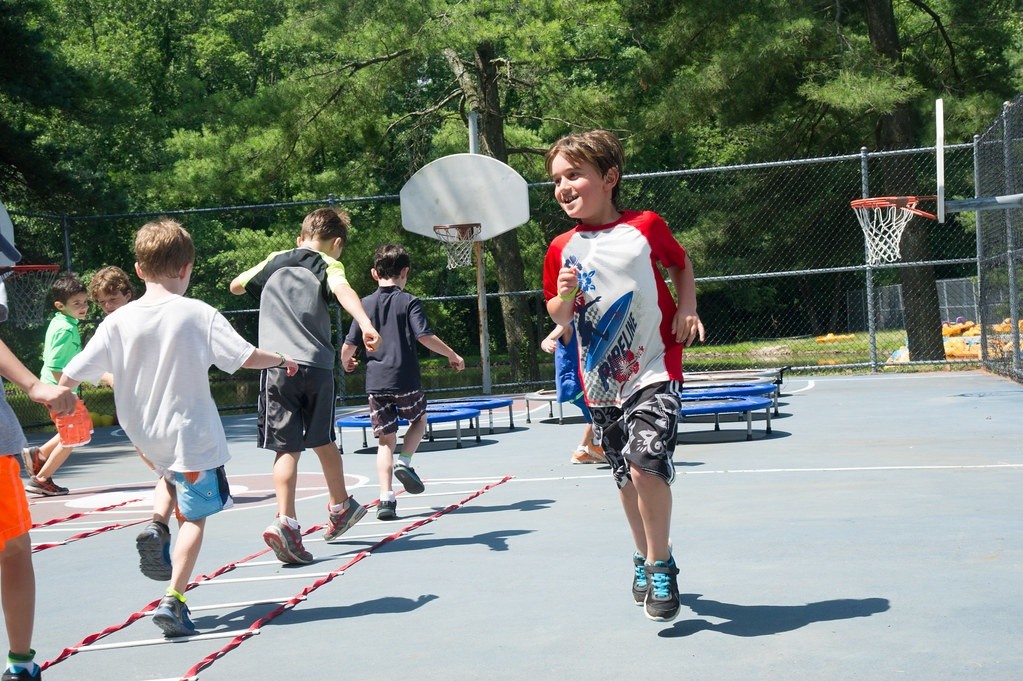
[
  {"xmin": 541, "ymin": 319, "xmax": 610, "ymax": 464},
  {"xmin": 21, "ymin": 272, "xmax": 93, "ymax": 497},
  {"xmin": 230, "ymin": 207, "xmax": 383, "ymax": 565},
  {"xmin": 87, "ymin": 265, "xmax": 188, "ymax": 531},
  {"xmin": 0, "ymin": 335, "xmax": 78, "ymax": 681},
  {"xmin": 57, "ymin": 220, "xmax": 299, "ymax": 635},
  {"xmin": 543, "ymin": 131, "xmax": 706, "ymax": 621},
  {"xmin": 341, "ymin": 243, "xmax": 465, "ymax": 521}
]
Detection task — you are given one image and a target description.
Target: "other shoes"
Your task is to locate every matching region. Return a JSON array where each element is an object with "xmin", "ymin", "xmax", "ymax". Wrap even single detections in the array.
[{"xmin": 3, "ymin": 664, "xmax": 44, "ymax": 680}]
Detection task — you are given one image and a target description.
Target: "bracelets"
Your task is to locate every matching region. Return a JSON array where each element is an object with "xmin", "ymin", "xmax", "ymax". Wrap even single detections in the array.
[
  {"xmin": 272, "ymin": 352, "xmax": 285, "ymax": 369},
  {"xmin": 557, "ymin": 280, "xmax": 579, "ymax": 302}
]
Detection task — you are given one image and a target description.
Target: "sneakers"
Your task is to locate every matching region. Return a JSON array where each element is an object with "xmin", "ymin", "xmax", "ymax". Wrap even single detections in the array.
[
  {"xmin": 263, "ymin": 516, "xmax": 314, "ymax": 564},
  {"xmin": 393, "ymin": 465, "xmax": 425, "ymax": 494},
  {"xmin": 135, "ymin": 523, "xmax": 174, "ymax": 581},
  {"xmin": 21, "ymin": 445, "xmax": 69, "ymax": 494},
  {"xmin": 572, "ymin": 439, "xmax": 607, "ymax": 463},
  {"xmin": 323, "ymin": 498, "xmax": 367, "ymax": 542},
  {"xmin": 377, "ymin": 498, "xmax": 398, "ymax": 519},
  {"xmin": 632, "ymin": 551, "xmax": 681, "ymax": 622},
  {"xmin": 152, "ymin": 596, "xmax": 195, "ymax": 636}
]
